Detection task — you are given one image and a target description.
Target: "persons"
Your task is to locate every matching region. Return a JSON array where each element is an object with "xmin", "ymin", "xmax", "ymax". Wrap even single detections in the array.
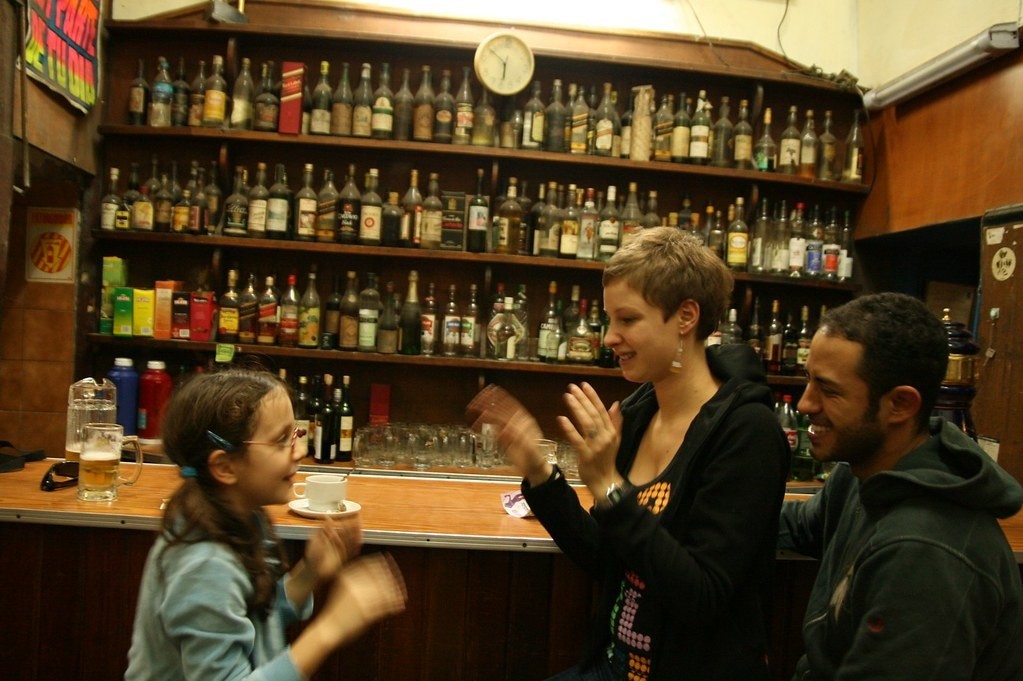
[
  {"xmin": 124, "ymin": 371, "xmax": 406, "ymax": 681},
  {"xmin": 467, "ymin": 227, "xmax": 795, "ymax": 681},
  {"xmin": 777, "ymin": 293, "xmax": 1023, "ymax": 681}
]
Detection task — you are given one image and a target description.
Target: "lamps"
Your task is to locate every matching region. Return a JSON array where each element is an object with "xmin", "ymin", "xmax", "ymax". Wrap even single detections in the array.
[{"xmin": 860, "ymin": 18, "xmax": 1021, "ymax": 110}]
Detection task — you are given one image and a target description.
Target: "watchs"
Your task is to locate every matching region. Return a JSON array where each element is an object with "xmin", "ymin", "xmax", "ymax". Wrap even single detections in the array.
[{"xmin": 595, "ymin": 479, "xmax": 632, "ymax": 509}]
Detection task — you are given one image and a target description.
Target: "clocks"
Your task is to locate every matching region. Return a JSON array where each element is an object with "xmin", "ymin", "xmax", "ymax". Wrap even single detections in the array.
[{"xmin": 473, "ymin": 30, "xmax": 537, "ymax": 96}]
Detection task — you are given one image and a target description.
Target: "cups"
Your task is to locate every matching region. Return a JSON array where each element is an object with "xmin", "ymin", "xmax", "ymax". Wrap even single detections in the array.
[
  {"xmin": 76, "ymin": 423, "xmax": 143, "ymax": 501},
  {"xmin": 351, "ymin": 419, "xmax": 581, "ymax": 478},
  {"xmin": 292, "ymin": 474, "xmax": 349, "ymax": 501}
]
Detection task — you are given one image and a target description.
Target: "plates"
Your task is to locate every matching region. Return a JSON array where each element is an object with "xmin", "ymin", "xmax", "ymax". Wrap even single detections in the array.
[{"xmin": 288, "ymin": 499, "xmax": 361, "ymax": 519}]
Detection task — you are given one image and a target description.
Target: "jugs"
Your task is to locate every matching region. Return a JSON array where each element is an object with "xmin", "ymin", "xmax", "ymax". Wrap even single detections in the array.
[{"xmin": 65, "ymin": 377, "xmax": 117, "ymax": 463}]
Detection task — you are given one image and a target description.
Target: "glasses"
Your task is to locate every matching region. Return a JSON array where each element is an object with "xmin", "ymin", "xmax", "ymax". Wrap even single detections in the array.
[
  {"xmin": 242, "ymin": 428, "xmax": 301, "ymax": 452},
  {"xmin": 39, "ymin": 460, "xmax": 80, "ymax": 492}
]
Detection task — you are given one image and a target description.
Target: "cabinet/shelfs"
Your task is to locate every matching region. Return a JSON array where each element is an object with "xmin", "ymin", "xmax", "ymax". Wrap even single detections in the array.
[{"xmin": 85, "ymin": 0, "xmax": 877, "ymax": 474}]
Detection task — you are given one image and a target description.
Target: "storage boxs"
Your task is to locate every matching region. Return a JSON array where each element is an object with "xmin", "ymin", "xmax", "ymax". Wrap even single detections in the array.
[
  {"xmin": 155, "ymin": 278, "xmax": 184, "ymax": 338},
  {"xmin": 439, "ymin": 189, "xmax": 467, "ymax": 250},
  {"xmin": 189, "ymin": 289, "xmax": 217, "ymax": 341},
  {"xmin": 278, "ymin": 58, "xmax": 311, "ymax": 136},
  {"xmin": 171, "ymin": 290, "xmax": 188, "ymax": 339},
  {"xmin": 132, "ymin": 288, "xmax": 156, "ymax": 336},
  {"xmin": 109, "ymin": 286, "xmax": 134, "ymax": 336},
  {"xmin": 98, "ymin": 255, "xmax": 129, "ymax": 334}
]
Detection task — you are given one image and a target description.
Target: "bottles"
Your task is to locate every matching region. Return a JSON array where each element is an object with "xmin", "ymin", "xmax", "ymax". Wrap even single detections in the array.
[
  {"xmin": 172, "ymin": 56, "xmax": 191, "ymax": 127},
  {"xmin": 138, "ymin": 360, "xmax": 173, "ymax": 439},
  {"xmin": 108, "ymin": 357, "xmax": 138, "ymax": 436},
  {"xmin": 99, "ymin": 140, "xmax": 854, "ymax": 291},
  {"xmin": 148, "ymin": 57, "xmax": 172, "ymax": 127},
  {"xmin": 176, "ymin": 258, "xmax": 852, "ymax": 386},
  {"xmin": 771, "ymin": 389, "xmax": 837, "ymax": 483},
  {"xmin": 278, "ymin": 366, "xmax": 354, "ymax": 463},
  {"xmin": 230, "ymin": 54, "xmax": 865, "ymax": 185},
  {"xmin": 128, "ymin": 59, "xmax": 149, "ymax": 125},
  {"xmin": 187, "ymin": 59, "xmax": 208, "ymax": 126},
  {"xmin": 202, "ymin": 55, "xmax": 227, "ymax": 128}
]
